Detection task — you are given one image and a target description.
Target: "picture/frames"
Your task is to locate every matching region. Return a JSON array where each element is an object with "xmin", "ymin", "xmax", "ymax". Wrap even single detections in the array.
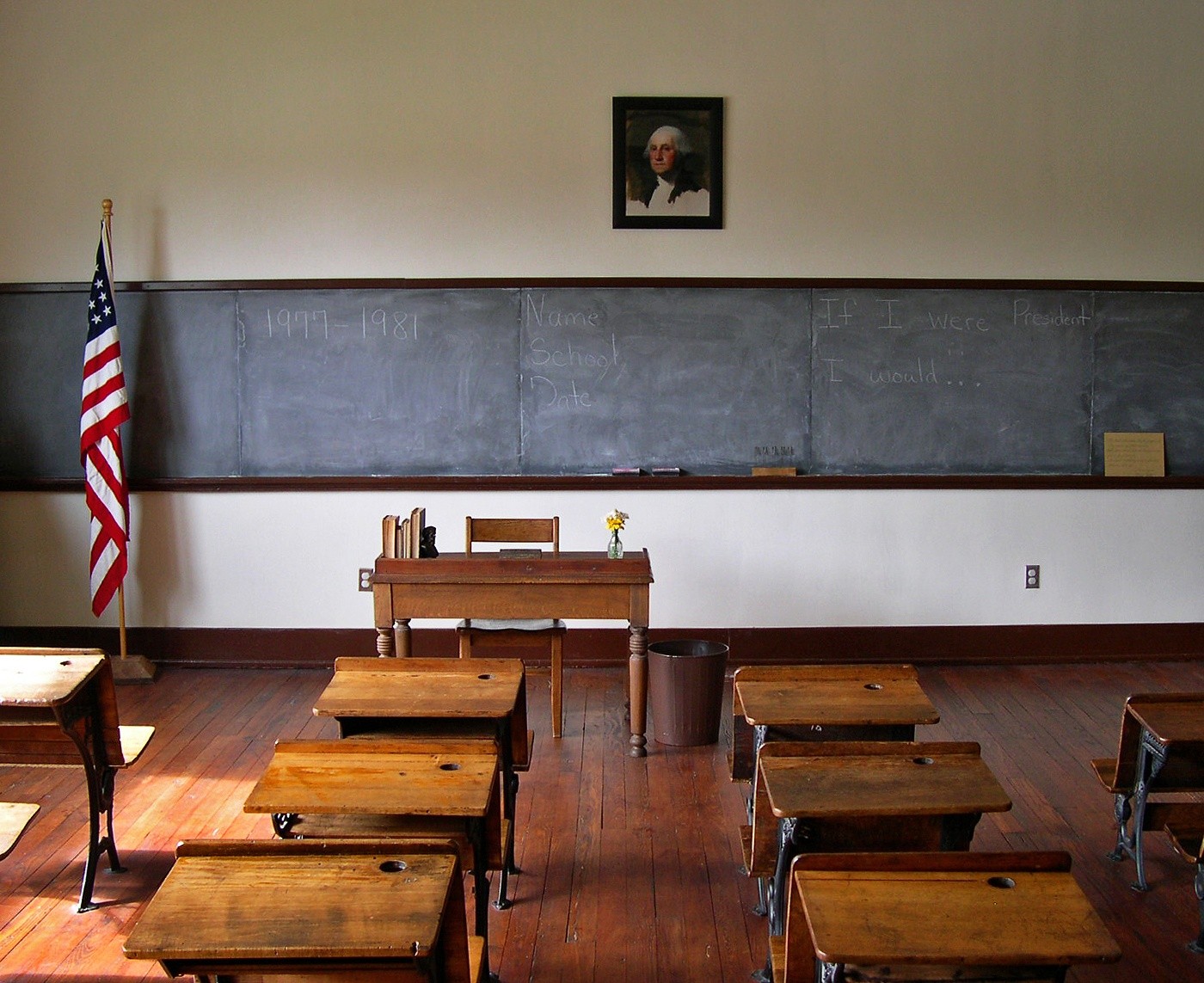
[{"xmin": 612, "ymin": 97, "xmax": 723, "ymax": 229}]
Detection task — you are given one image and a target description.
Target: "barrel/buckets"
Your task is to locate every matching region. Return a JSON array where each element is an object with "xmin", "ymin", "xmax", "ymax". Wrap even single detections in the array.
[{"xmin": 647, "ymin": 640, "xmax": 729, "ymax": 747}]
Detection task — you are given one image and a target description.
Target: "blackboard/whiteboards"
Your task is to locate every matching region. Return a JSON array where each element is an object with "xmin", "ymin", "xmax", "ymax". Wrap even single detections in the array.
[{"xmin": 0, "ymin": 278, "xmax": 1203, "ymax": 494}]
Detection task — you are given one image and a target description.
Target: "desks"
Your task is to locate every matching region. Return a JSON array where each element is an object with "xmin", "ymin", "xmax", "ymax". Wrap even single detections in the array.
[
  {"xmin": 724, "ymin": 663, "xmax": 1204, "ymax": 983},
  {"xmin": 0, "ymin": 547, "xmax": 655, "ymax": 983}
]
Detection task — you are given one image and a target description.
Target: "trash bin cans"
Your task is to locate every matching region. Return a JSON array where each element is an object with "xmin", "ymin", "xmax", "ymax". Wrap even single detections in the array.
[{"xmin": 646, "ymin": 639, "xmax": 728, "ymax": 746}]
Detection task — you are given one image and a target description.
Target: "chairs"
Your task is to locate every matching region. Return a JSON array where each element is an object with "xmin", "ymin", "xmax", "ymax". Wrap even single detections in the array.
[{"xmin": 455, "ymin": 516, "xmax": 567, "ymax": 738}]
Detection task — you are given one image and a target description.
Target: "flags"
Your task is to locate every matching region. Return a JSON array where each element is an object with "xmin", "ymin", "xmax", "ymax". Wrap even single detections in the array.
[{"xmin": 80, "ymin": 218, "xmax": 130, "ymax": 619}]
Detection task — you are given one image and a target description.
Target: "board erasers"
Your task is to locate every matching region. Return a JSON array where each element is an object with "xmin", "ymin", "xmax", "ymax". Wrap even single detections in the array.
[
  {"xmin": 650, "ymin": 468, "xmax": 681, "ymax": 478},
  {"xmin": 611, "ymin": 469, "xmax": 640, "ymax": 477}
]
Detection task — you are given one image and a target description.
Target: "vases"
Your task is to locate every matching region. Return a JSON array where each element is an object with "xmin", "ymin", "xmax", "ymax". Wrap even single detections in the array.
[{"xmin": 608, "ymin": 535, "xmax": 624, "ymax": 559}]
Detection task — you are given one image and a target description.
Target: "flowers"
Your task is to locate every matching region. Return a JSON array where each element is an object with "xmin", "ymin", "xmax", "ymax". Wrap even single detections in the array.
[{"xmin": 605, "ymin": 509, "xmax": 629, "ymax": 558}]
